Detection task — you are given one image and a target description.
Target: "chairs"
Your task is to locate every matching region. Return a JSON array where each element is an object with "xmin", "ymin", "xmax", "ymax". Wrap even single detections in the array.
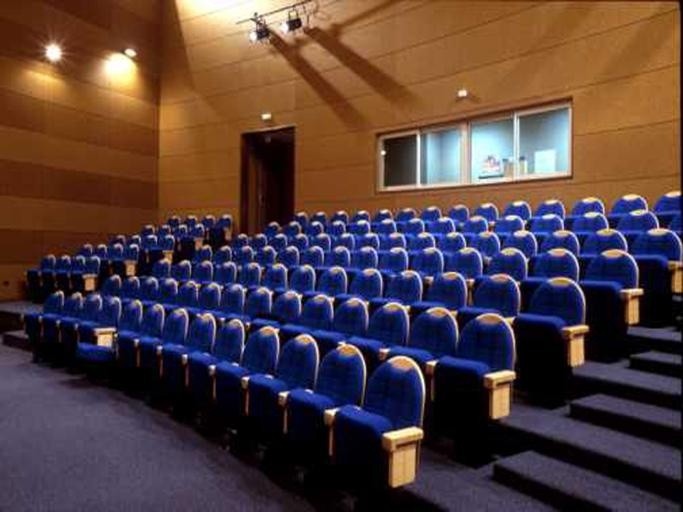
[{"xmin": 16, "ymin": 189, "xmax": 680, "ymax": 495}]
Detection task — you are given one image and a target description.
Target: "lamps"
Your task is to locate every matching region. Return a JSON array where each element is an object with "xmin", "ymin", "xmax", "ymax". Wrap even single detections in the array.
[{"xmin": 233, "ymin": 0, "xmax": 319, "ymax": 45}]
